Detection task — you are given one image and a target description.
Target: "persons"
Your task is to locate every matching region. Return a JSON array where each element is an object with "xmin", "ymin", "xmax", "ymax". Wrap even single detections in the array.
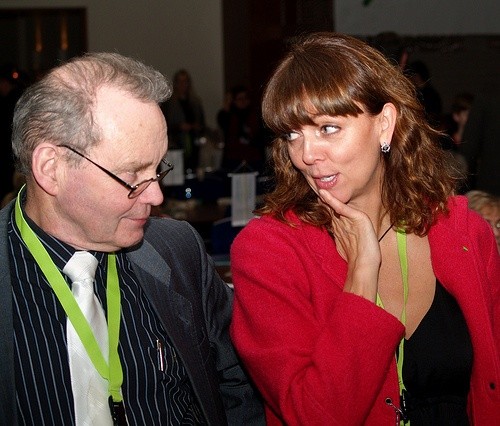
[
  {"xmin": 159, "ymin": 68, "xmax": 266, "ymax": 177},
  {"xmin": 371, "ymin": 33, "xmax": 500, "ymax": 257},
  {"xmin": 228, "ymin": 35, "xmax": 499, "ymax": 426},
  {"xmin": 0, "ymin": 53, "xmax": 267, "ymax": 426}
]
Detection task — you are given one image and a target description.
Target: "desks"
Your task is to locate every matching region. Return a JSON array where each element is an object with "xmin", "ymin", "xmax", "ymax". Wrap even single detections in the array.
[{"xmin": 160, "ymin": 171, "xmax": 230, "ymax": 225}]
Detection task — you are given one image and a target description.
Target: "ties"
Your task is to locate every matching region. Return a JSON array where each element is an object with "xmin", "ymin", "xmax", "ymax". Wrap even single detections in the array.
[{"xmin": 61, "ymin": 250, "xmax": 129, "ymax": 426}]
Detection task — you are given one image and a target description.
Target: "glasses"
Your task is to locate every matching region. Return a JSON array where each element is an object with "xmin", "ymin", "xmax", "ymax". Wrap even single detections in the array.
[{"xmin": 56, "ymin": 143, "xmax": 174, "ymax": 199}]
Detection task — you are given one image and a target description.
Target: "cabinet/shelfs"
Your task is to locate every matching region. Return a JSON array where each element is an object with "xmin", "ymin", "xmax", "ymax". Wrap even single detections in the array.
[{"xmin": 222, "ymin": 0, "xmax": 336, "ymax": 96}]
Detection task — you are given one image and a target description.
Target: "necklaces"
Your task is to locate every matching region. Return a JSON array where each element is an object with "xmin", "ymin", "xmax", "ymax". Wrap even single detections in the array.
[{"xmin": 378, "ymin": 215, "xmax": 404, "ymax": 242}]
